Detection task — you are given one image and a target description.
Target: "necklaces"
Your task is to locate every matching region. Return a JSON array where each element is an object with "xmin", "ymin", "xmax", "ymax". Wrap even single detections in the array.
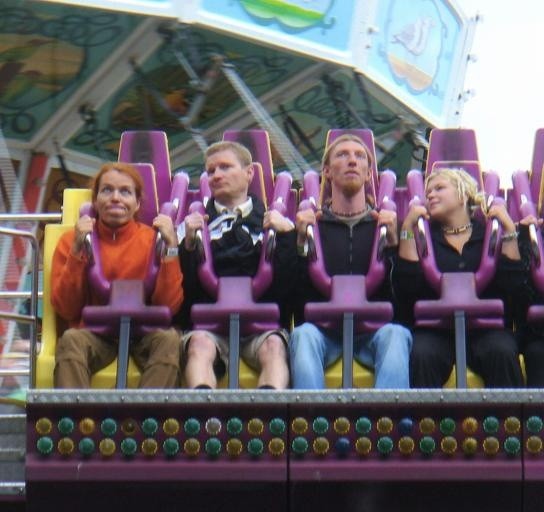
[{"xmin": 440, "ymin": 223, "xmax": 472, "ymax": 233}]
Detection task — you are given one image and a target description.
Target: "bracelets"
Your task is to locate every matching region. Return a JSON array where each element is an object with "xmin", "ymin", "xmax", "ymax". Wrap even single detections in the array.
[
  {"xmin": 398, "ymin": 230, "xmax": 415, "ymax": 240},
  {"xmin": 501, "ymin": 231, "xmax": 519, "ymax": 241},
  {"xmin": 163, "ymin": 246, "xmax": 180, "ymax": 257},
  {"xmin": 383, "ymin": 243, "xmax": 399, "ymax": 257},
  {"xmin": 295, "ymin": 241, "xmax": 309, "ymax": 255}
]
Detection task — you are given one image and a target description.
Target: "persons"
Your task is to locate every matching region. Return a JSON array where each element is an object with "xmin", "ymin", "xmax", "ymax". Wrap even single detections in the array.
[
  {"xmin": 177, "ymin": 139, "xmax": 308, "ymax": 389},
  {"xmin": 516, "ymin": 198, "xmax": 544, "ymax": 388},
  {"xmin": 395, "ymin": 164, "xmax": 531, "ymax": 388},
  {"xmin": 49, "ymin": 158, "xmax": 187, "ymax": 389},
  {"xmin": 289, "ymin": 133, "xmax": 414, "ymax": 389}
]
